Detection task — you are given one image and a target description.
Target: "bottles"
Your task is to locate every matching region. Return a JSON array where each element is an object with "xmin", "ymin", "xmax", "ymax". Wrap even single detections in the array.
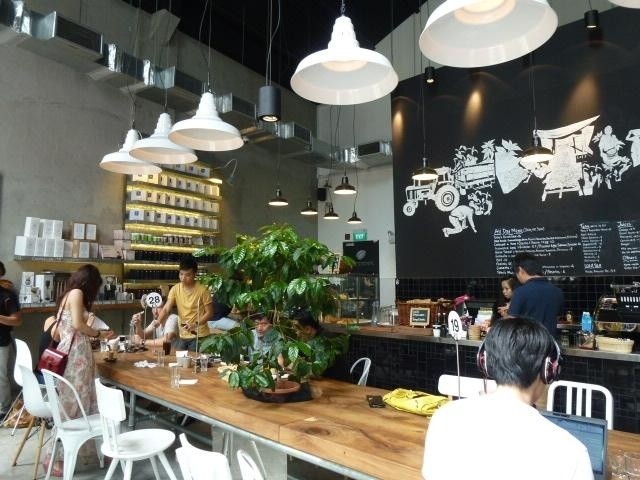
[
  {"xmin": 122, "ymin": 269, "xmax": 179, "ymax": 280},
  {"xmin": 431, "ymin": 324, "xmax": 441, "ymax": 337},
  {"xmin": 576, "ymin": 330, "xmax": 590, "ymax": 349},
  {"xmin": 435, "ymin": 313, "xmax": 444, "ymax": 337},
  {"xmin": 196, "ymin": 266, "xmax": 208, "ymax": 277},
  {"xmin": 441, "ymin": 312, "xmax": 448, "ymax": 336},
  {"xmin": 126, "ymin": 288, "xmax": 161, "ymax": 300},
  {"xmin": 116, "ymin": 277, "xmax": 122, "ymax": 293},
  {"xmin": 560, "ymin": 329, "xmax": 570, "ymax": 347},
  {"xmin": 104, "ymin": 278, "xmax": 116, "ymax": 300},
  {"xmin": 566, "ymin": 311, "xmax": 572, "ymax": 322},
  {"xmin": 135, "ymin": 249, "xmax": 192, "ymax": 262}
]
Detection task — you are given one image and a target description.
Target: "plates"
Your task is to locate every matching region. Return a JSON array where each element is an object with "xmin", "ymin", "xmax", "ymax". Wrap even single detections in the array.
[{"xmin": 104, "ymin": 358, "xmax": 116, "ymax": 362}]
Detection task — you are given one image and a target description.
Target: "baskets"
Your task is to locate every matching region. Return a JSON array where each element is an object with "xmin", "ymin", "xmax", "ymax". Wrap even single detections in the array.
[{"xmin": 396, "ymin": 300, "xmax": 439, "ymax": 325}]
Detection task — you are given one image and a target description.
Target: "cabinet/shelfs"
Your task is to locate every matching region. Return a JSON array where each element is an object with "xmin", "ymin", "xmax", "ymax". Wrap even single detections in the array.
[{"xmin": 123, "ymin": 168, "xmax": 223, "ymax": 282}]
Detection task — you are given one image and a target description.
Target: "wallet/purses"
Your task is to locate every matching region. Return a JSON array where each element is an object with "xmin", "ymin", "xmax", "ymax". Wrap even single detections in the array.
[{"xmin": 367, "ymin": 394, "xmax": 385, "ymax": 408}]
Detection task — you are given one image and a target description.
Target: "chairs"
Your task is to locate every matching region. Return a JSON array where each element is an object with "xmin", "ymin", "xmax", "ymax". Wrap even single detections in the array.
[
  {"xmin": 11, "ymin": 364, "xmax": 88, "ymax": 479},
  {"xmin": 0, "ymin": 338, "xmax": 57, "ymax": 436},
  {"xmin": 40, "ymin": 368, "xmax": 127, "ymax": 480}
]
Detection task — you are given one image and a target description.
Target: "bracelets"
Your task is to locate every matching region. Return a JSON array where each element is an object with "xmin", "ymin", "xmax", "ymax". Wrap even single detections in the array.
[
  {"xmin": 195, "ymin": 321, "xmax": 200, "ymax": 327},
  {"xmin": 94, "ymin": 330, "xmax": 101, "ymax": 339}
]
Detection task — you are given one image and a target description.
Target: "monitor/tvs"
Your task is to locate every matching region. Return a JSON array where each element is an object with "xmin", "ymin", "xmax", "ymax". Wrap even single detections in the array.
[{"xmin": 463, "ymin": 298, "xmax": 499, "ymax": 318}]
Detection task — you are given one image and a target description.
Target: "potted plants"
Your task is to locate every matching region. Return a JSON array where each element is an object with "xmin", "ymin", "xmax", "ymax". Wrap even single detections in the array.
[{"xmin": 192, "ymin": 222, "xmax": 361, "ymax": 401}]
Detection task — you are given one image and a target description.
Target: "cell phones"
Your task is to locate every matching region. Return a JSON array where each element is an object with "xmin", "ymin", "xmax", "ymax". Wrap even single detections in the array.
[{"xmin": 367, "ymin": 393, "xmax": 386, "ymax": 409}]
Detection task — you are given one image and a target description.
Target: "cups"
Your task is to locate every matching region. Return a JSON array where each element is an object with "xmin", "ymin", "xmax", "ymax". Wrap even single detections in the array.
[
  {"xmin": 199, "ymin": 357, "xmax": 208, "ymax": 372},
  {"xmin": 625, "ymin": 451, "xmax": 640, "ymax": 480},
  {"xmin": 170, "ymin": 365, "xmax": 183, "ymax": 389},
  {"xmin": 468, "ymin": 324, "xmax": 481, "ymax": 341},
  {"xmin": 608, "ymin": 449, "xmax": 630, "ymax": 478},
  {"xmin": 108, "ymin": 351, "xmax": 115, "ymax": 359},
  {"xmin": 156, "ymin": 350, "xmax": 165, "ymax": 366},
  {"xmin": 379, "ymin": 305, "xmax": 399, "ymax": 326}
]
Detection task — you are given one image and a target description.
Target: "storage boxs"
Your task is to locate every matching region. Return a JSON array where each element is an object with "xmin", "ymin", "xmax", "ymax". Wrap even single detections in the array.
[{"xmin": 12, "ymin": 217, "xmax": 98, "ymax": 258}]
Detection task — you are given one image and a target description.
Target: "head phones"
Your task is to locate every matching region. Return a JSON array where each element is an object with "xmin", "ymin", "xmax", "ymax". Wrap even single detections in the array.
[{"xmin": 476, "ymin": 315, "xmax": 563, "ymax": 386}]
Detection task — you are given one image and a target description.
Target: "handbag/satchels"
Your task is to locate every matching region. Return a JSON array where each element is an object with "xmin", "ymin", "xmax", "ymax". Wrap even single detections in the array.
[
  {"xmin": 37, "ymin": 348, "xmax": 68, "ymax": 376},
  {"xmin": 382, "ymin": 387, "xmax": 448, "ymax": 419}
]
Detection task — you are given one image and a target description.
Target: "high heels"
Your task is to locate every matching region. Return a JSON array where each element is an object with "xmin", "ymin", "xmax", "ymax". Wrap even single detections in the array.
[{"xmin": 44, "ymin": 459, "xmax": 63, "ymax": 476}]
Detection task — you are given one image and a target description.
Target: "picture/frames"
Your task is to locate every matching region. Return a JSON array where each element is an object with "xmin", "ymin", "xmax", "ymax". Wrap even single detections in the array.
[{"xmin": 409, "ymin": 308, "xmax": 430, "ymax": 326}]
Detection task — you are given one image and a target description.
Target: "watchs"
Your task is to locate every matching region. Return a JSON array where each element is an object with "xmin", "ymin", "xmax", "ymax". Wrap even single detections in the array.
[{"xmin": 140, "ymin": 338, "xmax": 147, "ymax": 346}]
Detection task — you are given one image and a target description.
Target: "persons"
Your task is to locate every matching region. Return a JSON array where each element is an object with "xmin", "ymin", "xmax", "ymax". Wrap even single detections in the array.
[
  {"xmin": 498, "ymin": 252, "xmax": 567, "ymax": 339},
  {"xmin": 35, "ymin": 294, "xmax": 65, "ymax": 395},
  {"xmin": 279, "ymin": 309, "xmax": 345, "ymax": 379},
  {"xmin": 487, "ymin": 274, "xmax": 521, "ymax": 327},
  {"xmin": 246, "ymin": 309, "xmax": 287, "ymax": 365},
  {"xmin": 420, "ymin": 317, "xmax": 593, "ymax": 480},
  {"xmin": 207, "ymin": 283, "xmax": 240, "ymax": 330},
  {"xmin": 130, "ymin": 297, "xmax": 178, "ymax": 345},
  {"xmin": 442, "ymin": 204, "xmax": 478, "ymax": 238},
  {"xmin": 0, "ymin": 262, "xmax": 22, "ymax": 420},
  {"xmin": 44, "ymin": 264, "xmax": 114, "ymax": 476},
  {"xmin": 152, "ymin": 257, "xmax": 213, "ymax": 352}
]
